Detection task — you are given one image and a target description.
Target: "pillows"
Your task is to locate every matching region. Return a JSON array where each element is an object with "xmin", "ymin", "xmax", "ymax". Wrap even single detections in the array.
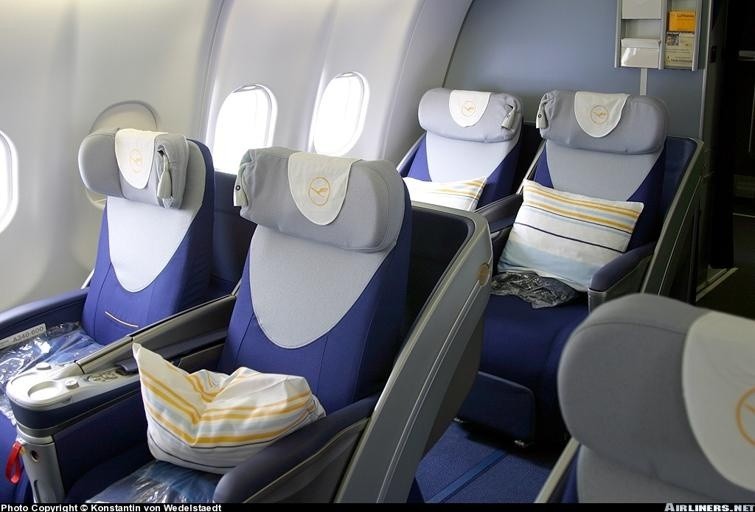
[
  {"xmin": 398, "ymin": 174, "xmax": 489, "ymax": 216},
  {"xmin": 495, "ymin": 176, "xmax": 645, "ymax": 293},
  {"xmin": 134, "ymin": 341, "xmax": 329, "ymax": 471}
]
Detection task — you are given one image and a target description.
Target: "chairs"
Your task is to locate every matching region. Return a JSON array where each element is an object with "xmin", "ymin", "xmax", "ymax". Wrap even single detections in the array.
[
  {"xmin": 396, "ymin": 86, "xmax": 541, "ymax": 219},
  {"xmin": 0, "ymin": 125, "xmax": 214, "ymax": 503},
  {"xmin": 555, "ymin": 291, "xmax": 755, "ymax": 503},
  {"xmin": 448, "ymin": 86, "xmax": 704, "ymax": 465},
  {"xmin": 56, "ymin": 144, "xmax": 411, "ymax": 503}
]
